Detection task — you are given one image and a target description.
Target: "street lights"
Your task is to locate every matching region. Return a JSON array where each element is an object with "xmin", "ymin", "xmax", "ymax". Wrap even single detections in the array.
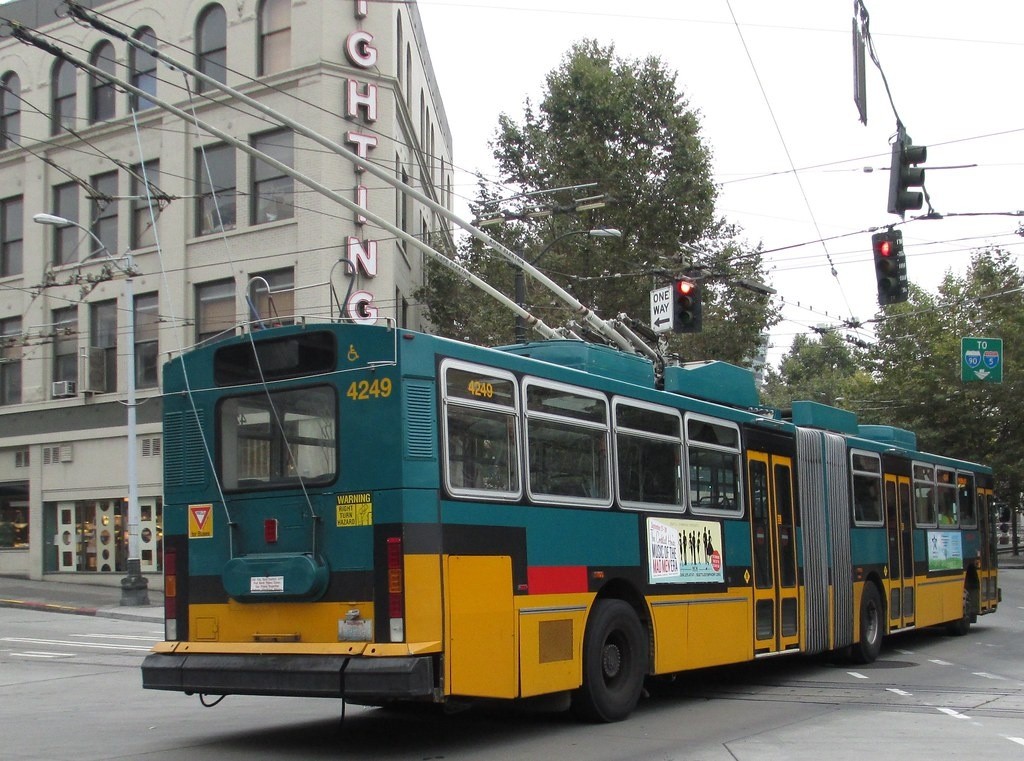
[
  {"xmin": 508, "ymin": 226, "xmax": 620, "ymax": 343},
  {"xmin": 33, "ymin": 212, "xmax": 150, "ymax": 607}
]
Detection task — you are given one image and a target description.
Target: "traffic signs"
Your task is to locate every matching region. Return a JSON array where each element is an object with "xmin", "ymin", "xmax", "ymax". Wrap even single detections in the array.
[
  {"xmin": 959, "ymin": 336, "xmax": 1005, "ymax": 385},
  {"xmin": 649, "ymin": 285, "xmax": 673, "ymax": 333}
]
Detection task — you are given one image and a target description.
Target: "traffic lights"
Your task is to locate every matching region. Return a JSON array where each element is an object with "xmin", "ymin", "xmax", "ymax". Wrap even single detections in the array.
[
  {"xmin": 889, "ymin": 140, "xmax": 928, "ymax": 219},
  {"xmin": 673, "ymin": 275, "xmax": 706, "ymax": 335},
  {"xmin": 872, "ymin": 230, "xmax": 910, "ymax": 306}
]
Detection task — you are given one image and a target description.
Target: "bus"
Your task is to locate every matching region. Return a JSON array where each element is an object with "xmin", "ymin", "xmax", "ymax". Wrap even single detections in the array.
[{"xmin": 3, "ymin": 0, "xmax": 1010, "ymax": 724}]
